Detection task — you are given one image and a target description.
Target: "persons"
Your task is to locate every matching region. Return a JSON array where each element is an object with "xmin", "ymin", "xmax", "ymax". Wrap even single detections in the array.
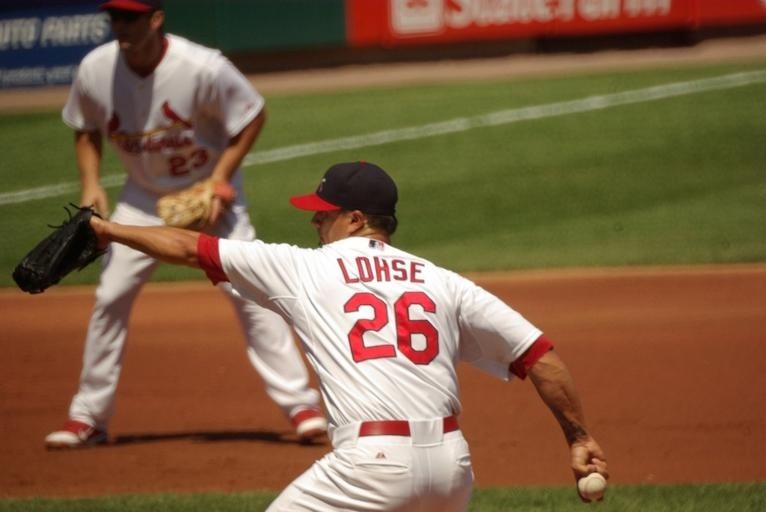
[
  {"xmin": 11, "ymin": 159, "xmax": 611, "ymax": 511},
  {"xmin": 43, "ymin": 0, "xmax": 329, "ymax": 450}
]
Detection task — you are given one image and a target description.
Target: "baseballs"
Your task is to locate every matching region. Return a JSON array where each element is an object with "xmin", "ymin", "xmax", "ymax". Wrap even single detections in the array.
[{"xmin": 578, "ymin": 473, "xmax": 606, "ymax": 500}]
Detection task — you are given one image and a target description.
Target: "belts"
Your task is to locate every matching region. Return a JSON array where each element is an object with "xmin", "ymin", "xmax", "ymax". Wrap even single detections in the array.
[{"xmin": 358, "ymin": 415, "xmax": 460, "ymax": 437}]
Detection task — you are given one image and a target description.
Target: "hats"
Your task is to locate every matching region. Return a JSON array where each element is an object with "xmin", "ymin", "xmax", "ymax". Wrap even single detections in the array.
[
  {"xmin": 95, "ymin": 0, "xmax": 163, "ymax": 16},
  {"xmin": 288, "ymin": 159, "xmax": 399, "ymax": 216}
]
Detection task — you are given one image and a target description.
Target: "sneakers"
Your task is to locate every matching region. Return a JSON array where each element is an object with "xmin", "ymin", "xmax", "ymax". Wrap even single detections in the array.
[
  {"xmin": 288, "ymin": 404, "xmax": 328, "ymax": 442},
  {"xmin": 43, "ymin": 418, "xmax": 108, "ymax": 450}
]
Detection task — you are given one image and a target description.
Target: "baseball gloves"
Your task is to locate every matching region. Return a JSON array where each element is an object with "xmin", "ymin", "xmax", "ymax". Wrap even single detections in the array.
[
  {"xmin": 156, "ymin": 178, "xmax": 237, "ymax": 227},
  {"xmin": 12, "ymin": 207, "xmax": 103, "ymax": 294}
]
